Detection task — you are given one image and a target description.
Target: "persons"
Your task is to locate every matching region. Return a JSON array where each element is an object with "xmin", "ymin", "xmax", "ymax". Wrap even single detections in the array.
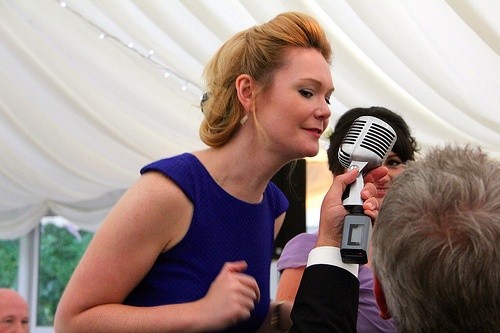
[
  {"xmin": 273, "ymin": 105, "xmax": 416, "ymax": 333},
  {"xmin": 53, "ymin": 11, "xmax": 335, "ymax": 333},
  {"xmin": 290, "ymin": 146, "xmax": 500, "ymax": 333},
  {"xmin": 0, "ymin": 288, "xmax": 30, "ymax": 333}
]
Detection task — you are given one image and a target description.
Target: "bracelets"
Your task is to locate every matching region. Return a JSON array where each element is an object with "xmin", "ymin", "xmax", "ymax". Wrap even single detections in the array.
[{"xmin": 269, "ymin": 301, "xmax": 283, "ymax": 333}]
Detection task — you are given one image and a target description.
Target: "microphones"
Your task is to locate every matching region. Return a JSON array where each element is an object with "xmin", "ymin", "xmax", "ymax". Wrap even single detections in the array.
[{"xmin": 336, "ymin": 112, "xmax": 400, "ymax": 213}]
